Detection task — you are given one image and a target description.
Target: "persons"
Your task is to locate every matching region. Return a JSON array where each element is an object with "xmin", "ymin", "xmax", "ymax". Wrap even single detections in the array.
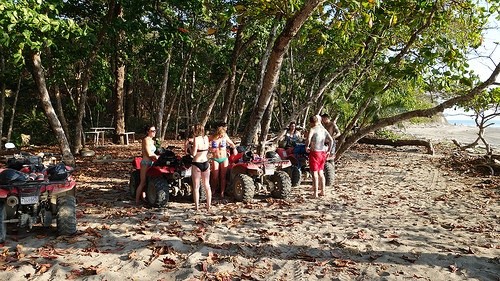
[
  {"xmin": 134, "ymin": 124, "xmax": 159, "ymax": 206},
  {"xmin": 186, "ymin": 123, "xmax": 212, "ymax": 211},
  {"xmin": 208, "ymin": 120, "xmax": 239, "ymax": 200},
  {"xmin": 276, "ymin": 120, "xmax": 303, "ymax": 147},
  {"xmin": 305, "ymin": 114, "xmax": 333, "ymax": 199},
  {"xmin": 318, "ymin": 113, "xmax": 341, "ymax": 186}
]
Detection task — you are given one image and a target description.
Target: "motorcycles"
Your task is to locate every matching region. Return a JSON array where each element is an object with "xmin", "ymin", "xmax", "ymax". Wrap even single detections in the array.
[
  {"xmin": 129, "ymin": 145, "xmax": 193, "ymax": 207},
  {"xmin": 276, "ymin": 142, "xmax": 335, "ymax": 186},
  {"xmin": 0, "ymin": 152, "xmax": 76, "ymax": 243},
  {"xmin": 226, "ymin": 146, "xmax": 292, "ymax": 203}
]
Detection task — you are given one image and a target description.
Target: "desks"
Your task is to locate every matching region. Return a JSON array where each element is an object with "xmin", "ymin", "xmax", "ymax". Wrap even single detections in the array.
[{"xmin": 90, "ymin": 127, "xmax": 115, "ymax": 146}]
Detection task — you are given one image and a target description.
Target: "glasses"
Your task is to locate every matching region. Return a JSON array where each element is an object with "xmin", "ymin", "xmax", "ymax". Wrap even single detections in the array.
[{"xmin": 149, "ymin": 130, "xmax": 156, "ymax": 132}]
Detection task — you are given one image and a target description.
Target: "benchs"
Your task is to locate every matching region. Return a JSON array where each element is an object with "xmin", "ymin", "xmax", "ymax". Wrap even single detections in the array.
[
  {"xmin": 123, "ymin": 131, "xmax": 135, "ymax": 144},
  {"xmin": 84, "ymin": 130, "xmax": 105, "ymax": 146}
]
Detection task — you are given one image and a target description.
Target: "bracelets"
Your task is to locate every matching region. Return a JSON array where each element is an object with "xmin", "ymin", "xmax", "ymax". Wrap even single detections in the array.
[
  {"xmin": 233, "ymin": 146, "xmax": 236, "ymax": 149},
  {"xmin": 305, "ymin": 145, "xmax": 309, "ymax": 147}
]
